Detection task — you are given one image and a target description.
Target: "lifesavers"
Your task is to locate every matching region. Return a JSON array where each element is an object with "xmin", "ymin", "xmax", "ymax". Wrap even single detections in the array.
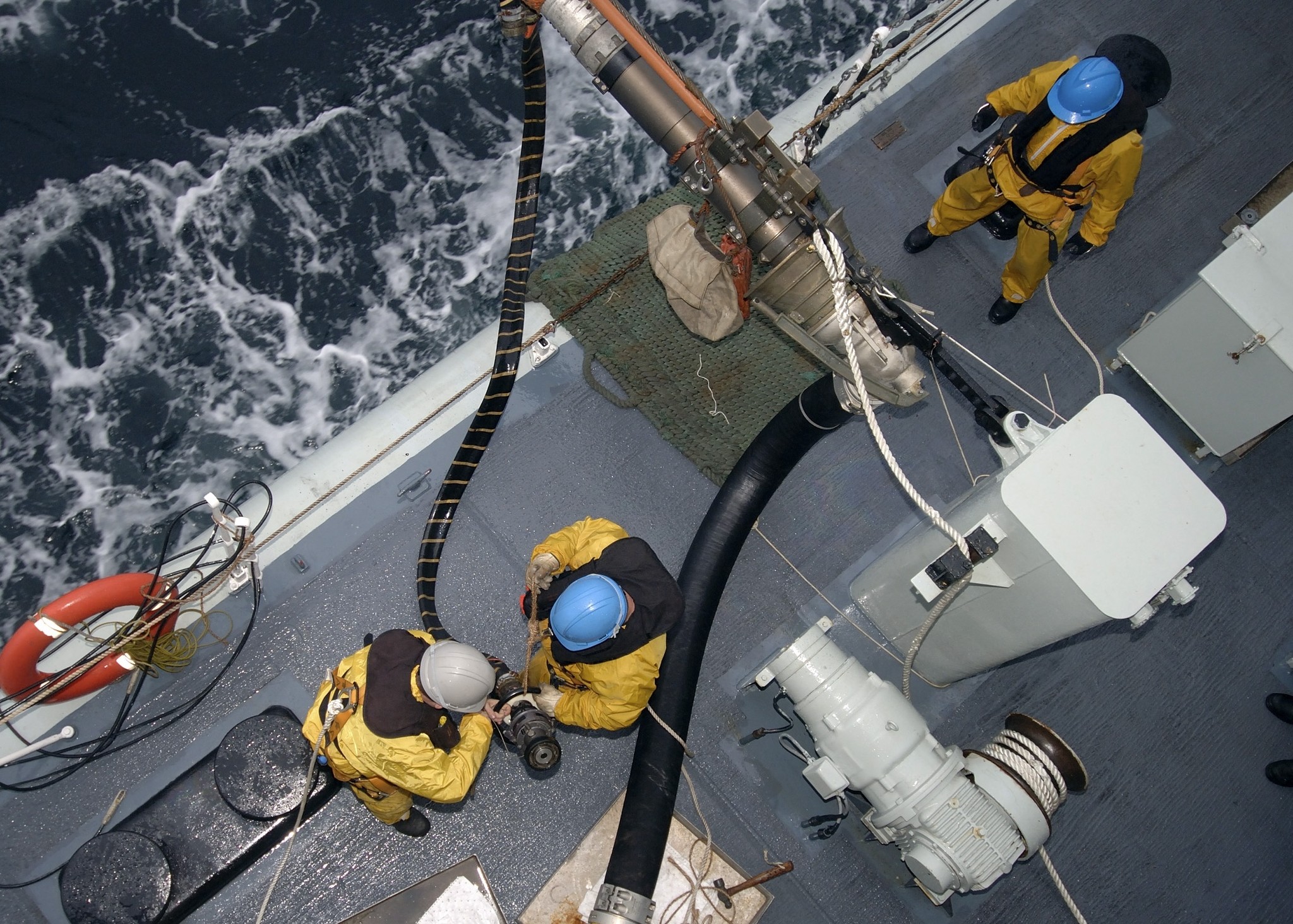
[{"xmin": 1, "ymin": 571, "xmax": 182, "ymax": 706}]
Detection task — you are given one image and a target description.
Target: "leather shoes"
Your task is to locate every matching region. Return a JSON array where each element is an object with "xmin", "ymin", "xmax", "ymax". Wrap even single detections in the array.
[
  {"xmin": 903, "ymin": 220, "xmax": 938, "ymax": 254},
  {"xmin": 1265, "ymin": 760, "xmax": 1293, "ymax": 788},
  {"xmin": 1266, "ymin": 693, "xmax": 1293, "ymax": 725},
  {"xmin": 988, "ymin": 294, "xmax": 1023, "ymax": 324}
]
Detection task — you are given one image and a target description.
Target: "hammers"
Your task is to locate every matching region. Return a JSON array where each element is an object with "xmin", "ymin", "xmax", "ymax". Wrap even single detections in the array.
[{"xmin": 713, "ymin": 862, "xmax": 793, "ymax": 909}]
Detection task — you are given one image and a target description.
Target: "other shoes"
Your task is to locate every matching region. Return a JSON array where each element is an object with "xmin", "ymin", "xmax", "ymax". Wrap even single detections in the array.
[{"xmin": 392, "ymin": 807, "xmax": 431, "ymax": 838}]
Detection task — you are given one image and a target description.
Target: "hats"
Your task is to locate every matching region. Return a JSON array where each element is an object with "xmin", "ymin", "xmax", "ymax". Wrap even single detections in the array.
[{"xmin": 1048, "ymin": 57, "xmax": 1124, "ymax": 124}]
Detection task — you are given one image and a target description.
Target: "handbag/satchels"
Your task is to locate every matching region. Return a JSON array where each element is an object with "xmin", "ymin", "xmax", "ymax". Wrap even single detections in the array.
[{"xmin": 645, "ymin": 205, "xmax": 744, "ymax": 342}]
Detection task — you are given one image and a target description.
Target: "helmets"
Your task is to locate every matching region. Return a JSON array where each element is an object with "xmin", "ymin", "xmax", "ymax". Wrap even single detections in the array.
[
  {"xmin": 420, "ymin": 641, "xmax": 496, "ymax": 713},
  {"xmin": 550, "ymin": 573, "xmax": 628, "ymax": 652}
]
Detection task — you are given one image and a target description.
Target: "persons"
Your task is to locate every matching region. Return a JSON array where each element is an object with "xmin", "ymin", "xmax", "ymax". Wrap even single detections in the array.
[
  {"xmin": 508, "ymin": 513, "xmax": 680, "ymax": 730},
  {"xmin": 301, "ymin": 627, "xmax": 506, "ymax": 835},
  {"xmin": 904, "ymin": 55, "xmax": 1144, "ymax": 326}
]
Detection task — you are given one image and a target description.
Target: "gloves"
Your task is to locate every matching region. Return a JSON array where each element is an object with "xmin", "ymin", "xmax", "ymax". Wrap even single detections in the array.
[
  {"xmin": 532, "ymin": 681, "xmax": 564, "ymax": 717},
  {"xmin": 526, "ymin": 553, "xmax": 558, "ymax": 595},
  {"xmin": 1062, "ymin": 230, "xmax": 1093, "ymax": 256},
  {"xmin": 972, "ymin": 102, "xmax": 999, "ymax": 132}
]
